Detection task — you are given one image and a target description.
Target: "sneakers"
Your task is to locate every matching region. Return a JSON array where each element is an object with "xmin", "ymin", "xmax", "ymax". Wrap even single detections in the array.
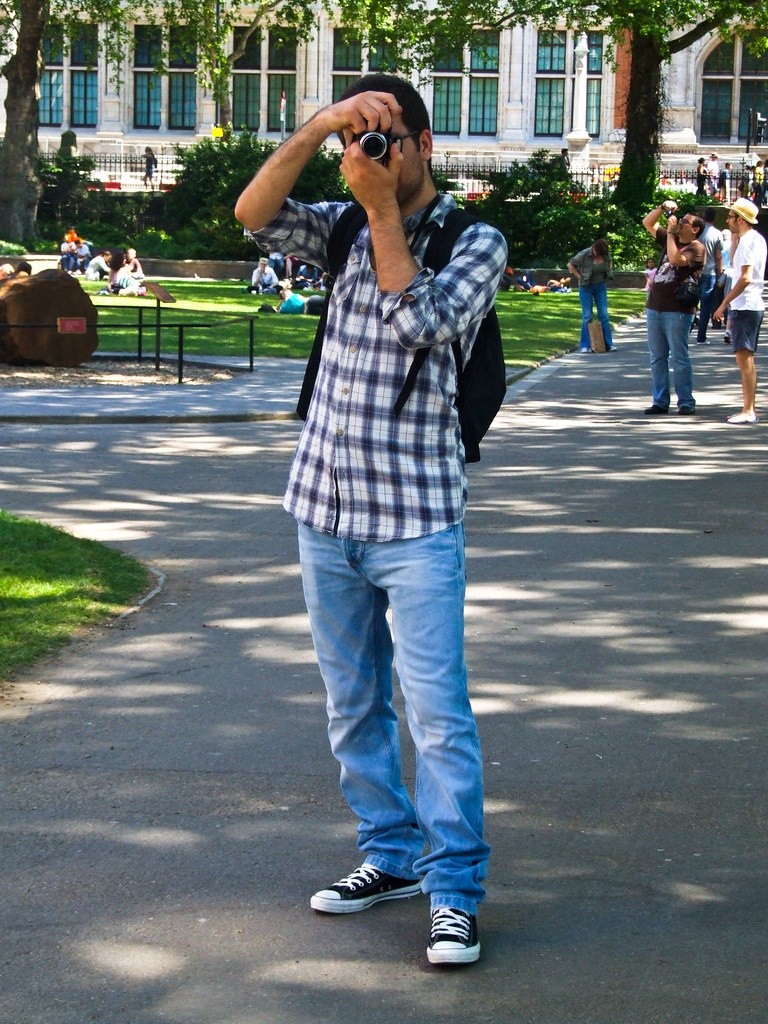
[
  {"xmin": 427, "ymin": 905, "xmax": 481, "ymax": 963},
  {"xmin": 310, "ymin": 865, "xmax": 422, "ymax": 912}
]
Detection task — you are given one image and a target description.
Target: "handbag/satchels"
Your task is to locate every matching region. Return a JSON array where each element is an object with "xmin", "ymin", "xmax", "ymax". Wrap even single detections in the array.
[
  {"xmin": 676, "ymin": 282, "xmax": 702, "ymax": 306},
  {"xmin": 588, "ymin": 319, "xmax": 606, "ymax": 353}
]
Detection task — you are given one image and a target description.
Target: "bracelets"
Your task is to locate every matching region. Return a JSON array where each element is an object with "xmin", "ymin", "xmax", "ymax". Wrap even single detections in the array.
[
  {"xmin": 668, "ymin": 231, "xmax": 675, "ymax": 236},
  {"xmin": 661, "ymin": 203, "xmax": 666, "ymax": 211}
]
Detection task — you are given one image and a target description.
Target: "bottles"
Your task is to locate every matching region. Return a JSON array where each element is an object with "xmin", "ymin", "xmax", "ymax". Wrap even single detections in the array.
[{"xmin": 259, "ymin": 284, "xmax": 262, "ymax": 296}]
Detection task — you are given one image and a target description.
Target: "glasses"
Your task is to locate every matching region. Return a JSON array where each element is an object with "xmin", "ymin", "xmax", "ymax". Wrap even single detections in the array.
[
  {"xmin": 387, "ymin": 130, "xmax": 419, "ymax": 154},
  {"xmin": 714, "ymin": 156, "xmax": 718, "ymax": 158},
  {"xmin": 726, "ymin": 214, "xmax": 739, "ymax": 220},
  {"xmin": 678, "ymin": 219, "xmax": 692, "ymax": 226}
]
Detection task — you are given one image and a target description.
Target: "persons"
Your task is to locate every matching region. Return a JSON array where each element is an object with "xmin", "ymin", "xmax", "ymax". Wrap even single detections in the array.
[
  {"xmin": 235, "ymin": 75, "xmax": 508, "ymax": 964},
  {"xmin": 690, "ymin": 207, "xmax": 740, "ymax": 345},
  {"xmin": 641, "ymin": 201, "xmax": 707, "ymax": 416},
  {"xmin": 0, "ymin": 262, "xmax": 32, "ymax": 279},
  {"xmin": 60, "ymin": 225, "xmax": 146, "ymax": 297},
  {"xmin": 713, "ymin": 197, "xmax": 766, "ymax": 423},
  {"xmin": 567, "ymin": 239, "xmax": 617, "ymax": 353},
  {"xmin": 502, "ymin": 265, "xmax": 572, "ymax": 293},
  {"xmin": 644, "ymin": 258, "xmax": 658, "ymax": 298},
  {"xmin": 142, "ymin": 146, "xmax": 155, "ymax": 191},
  {"xmin": 246, "ymin": 244, "xmax": 328, "ymax": 316},
  {"xmin": 696, "ymin": 151, "xmax": 768, "ymax": 208}
]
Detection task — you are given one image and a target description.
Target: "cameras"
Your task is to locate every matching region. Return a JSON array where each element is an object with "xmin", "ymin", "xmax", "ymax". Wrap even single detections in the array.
[
  {"xmin": 666, "ymin": 209, "xmax": 673, "ymax": 219},
  {"xmin": 351, "ymin": 125, "xmax": 394, "ymax": 169}
]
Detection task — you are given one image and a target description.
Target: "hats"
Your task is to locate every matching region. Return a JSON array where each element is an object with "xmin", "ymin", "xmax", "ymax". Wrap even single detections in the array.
[{"xmin": 725, "ymin": 198, "xmax": 759, "ymax": 224}]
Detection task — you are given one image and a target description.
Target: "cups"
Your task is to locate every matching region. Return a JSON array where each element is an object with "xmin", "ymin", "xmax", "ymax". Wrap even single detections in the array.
[{"xmin": 251, "ymin": 290, "xmax": 256, "ymax": 294}]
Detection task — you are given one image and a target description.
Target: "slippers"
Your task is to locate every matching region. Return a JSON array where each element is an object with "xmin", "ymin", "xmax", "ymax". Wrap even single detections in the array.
[{"xmin": 726, "ymin": 412, "xmax": 759, "ymax": 423}]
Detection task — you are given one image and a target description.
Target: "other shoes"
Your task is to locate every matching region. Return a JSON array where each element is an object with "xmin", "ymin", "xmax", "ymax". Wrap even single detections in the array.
[
  {"xmin": 723, "ymin": 333, "xmax": 731, "ymax": 344},
  {"xmin": 710, "ymin": 321, "xmax": 721, "ymax": 329},
  {"xmin": 644, "ymin": 404, "xmax": 668, "ymax": 415},
  {"xmin": 697, "ymin": 339, "xmax": 712, "ymax": 345},
  {"xmin": 607, "ymin": 346, "xmax": 617, "ymax": 350},
  {"xmin": 678, "ymin": 405, "xmax": 695, "ymax": 414},
  {"xmin": 693, "ymin": 318, "xmax": 700, "ymax": 326},
  {"xmin": 581, "ymin": 346, "xmax": 587, "ymax": 353}
]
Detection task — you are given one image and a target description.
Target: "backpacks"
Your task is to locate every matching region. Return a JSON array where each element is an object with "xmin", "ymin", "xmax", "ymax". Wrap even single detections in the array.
[{"xmin": 296, "ymin": 200, "xmax": 507, "ymax": 463}]
